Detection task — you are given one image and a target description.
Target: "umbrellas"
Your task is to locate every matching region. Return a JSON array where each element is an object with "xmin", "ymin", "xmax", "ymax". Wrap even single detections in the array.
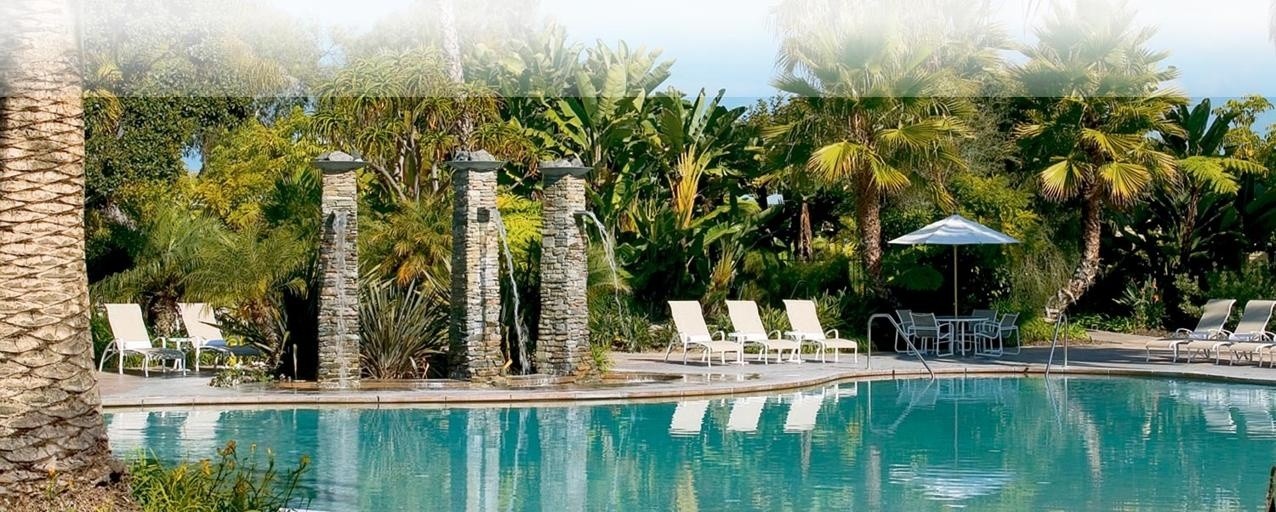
[{"xmin": 887, "ymin": 215, "xmax": 1019, "ymax": 350}]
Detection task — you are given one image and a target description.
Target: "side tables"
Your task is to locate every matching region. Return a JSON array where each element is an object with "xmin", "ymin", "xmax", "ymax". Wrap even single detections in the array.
[
  {"xmin": 728, "ymin": 332, "xmax": 760, "ymax": 365},
  {"xmin": 784, "ymin": 331, "xmax": 813, "ymax": 362},
  {"xmin": 167, "ymin": 338, "xmax": 193, "ymax": 372}
]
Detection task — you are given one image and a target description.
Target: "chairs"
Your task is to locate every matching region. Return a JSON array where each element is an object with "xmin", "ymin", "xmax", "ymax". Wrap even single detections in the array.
[
  {"xmin": 724, "ymin": 299, "xmax": 802, "ymax": 365},
  {"xmin": 1145, "ymin": 298, "xmax": 1276, "ymax": 367},
  {"xmin": 895, "ymin": 377, "xmax": 1021, "ymax": 410},
  {"xmin": 98, "ymin": 304, "xmax": 187, "ymax": 378},
  {"xmin": 893, "ymin": 308, "xmax": 1021, "ymax": 357},
  {"xmin": 1173, "ymin": 384, "xmax": 1275, "ymax": 441},
  {"xmin": 782, "ymin": 299, "xmax": 858, "ymax": 364},
  {"xmin": 664, "ymin": 300, "xmax": 745, "ymax": 368},
  {"xmin": 668, "ymin": 381, "xmax": 861, "ymax": 438},
  {"xmin": 177, "ymin": 302, "xmax": 261, "ymax": 372}
]
